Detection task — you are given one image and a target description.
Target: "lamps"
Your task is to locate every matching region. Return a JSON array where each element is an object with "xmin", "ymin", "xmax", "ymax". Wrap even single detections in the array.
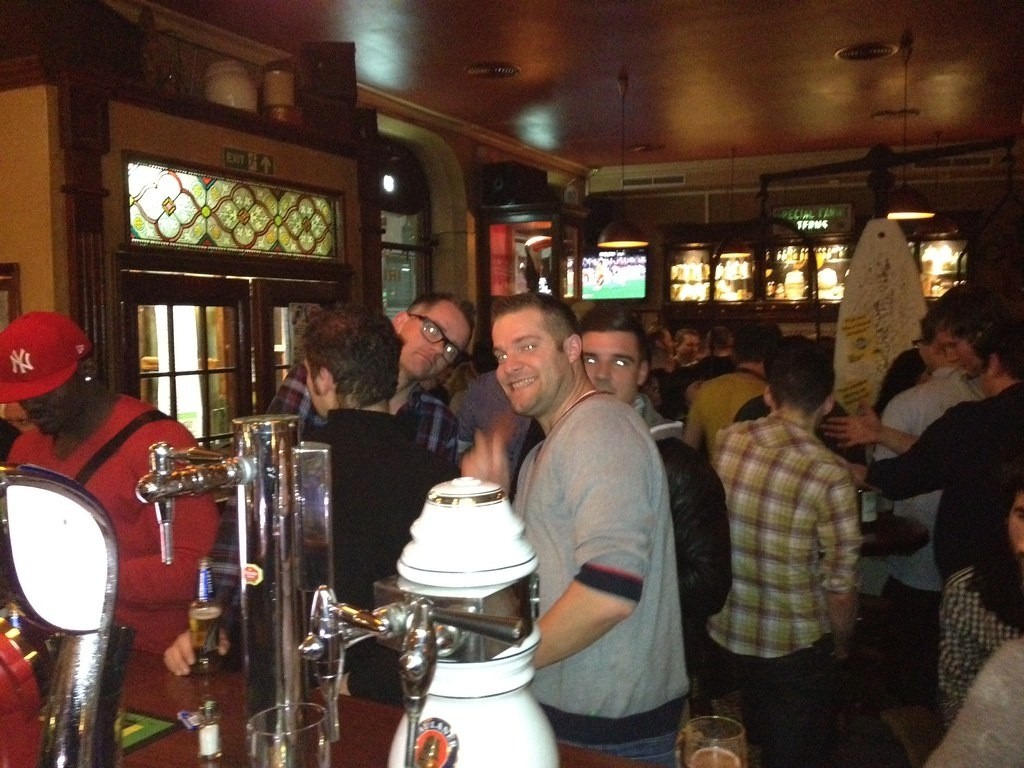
[
  {"xmin": 597, "ymin": 71, "xmax": 651, "ymax": 249},
  {"xmin": 883, "ymin": 34, "xmax": 937, "ymax": 221}
]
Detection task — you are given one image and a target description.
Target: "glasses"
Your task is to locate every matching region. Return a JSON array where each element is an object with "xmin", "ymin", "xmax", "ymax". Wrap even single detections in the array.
[{"xmin": 409, "ymin": 314, "xmax": 466, "ymax": 366}]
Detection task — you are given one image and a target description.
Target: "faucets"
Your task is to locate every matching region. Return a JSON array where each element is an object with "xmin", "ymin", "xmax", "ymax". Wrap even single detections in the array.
[
  {"xmin": 136, "ymin": 440, "xmax": 228, "ymax": 577},
  {"xmin": 399, "ymin": 601, "xmax": 526, "ymax": 721}
]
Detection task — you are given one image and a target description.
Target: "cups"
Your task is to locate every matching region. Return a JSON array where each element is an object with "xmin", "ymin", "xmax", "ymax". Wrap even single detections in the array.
[
  {"xmin": 676, "ymin": 714, "xmax": 750, "ymax": 768},
  {"xmin": 244, "ymin": 703, "xmax": 332, "ymax": 768}
]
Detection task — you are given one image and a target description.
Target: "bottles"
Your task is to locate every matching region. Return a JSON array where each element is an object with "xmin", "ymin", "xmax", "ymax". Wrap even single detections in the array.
[
  {"xmin": 3, "ymin": 603, "xmax": 25, "ymax": 632},
  {"xmin": 187, "ymin": 557, "xmax": 224, "ymax": 681},
  {"xmin": 857, "ymin": 486, "xmax": 880, "ymax": 536},
  {"xmin": 198, "ymin": 695, "xmax": 223, "ymax": 762}
]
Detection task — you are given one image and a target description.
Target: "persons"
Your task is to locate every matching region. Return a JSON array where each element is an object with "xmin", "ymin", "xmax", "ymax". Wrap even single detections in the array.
[
  {"xmin": 0, "ymin": 309, "xmax": 218, "ymax": 660},
  {"xmin": 163, "ymin": 281, "xmax": 1024, "ymax": 766},
  {"xmin": 1, "ymin": 400, "xmax": 36, "ymax": 435}
]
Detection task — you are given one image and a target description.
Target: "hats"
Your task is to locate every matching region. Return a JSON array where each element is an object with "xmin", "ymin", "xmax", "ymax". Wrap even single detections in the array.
[{"xmin": 0, "ymin": 312, "xmax": 92, "ymax": 404}]
